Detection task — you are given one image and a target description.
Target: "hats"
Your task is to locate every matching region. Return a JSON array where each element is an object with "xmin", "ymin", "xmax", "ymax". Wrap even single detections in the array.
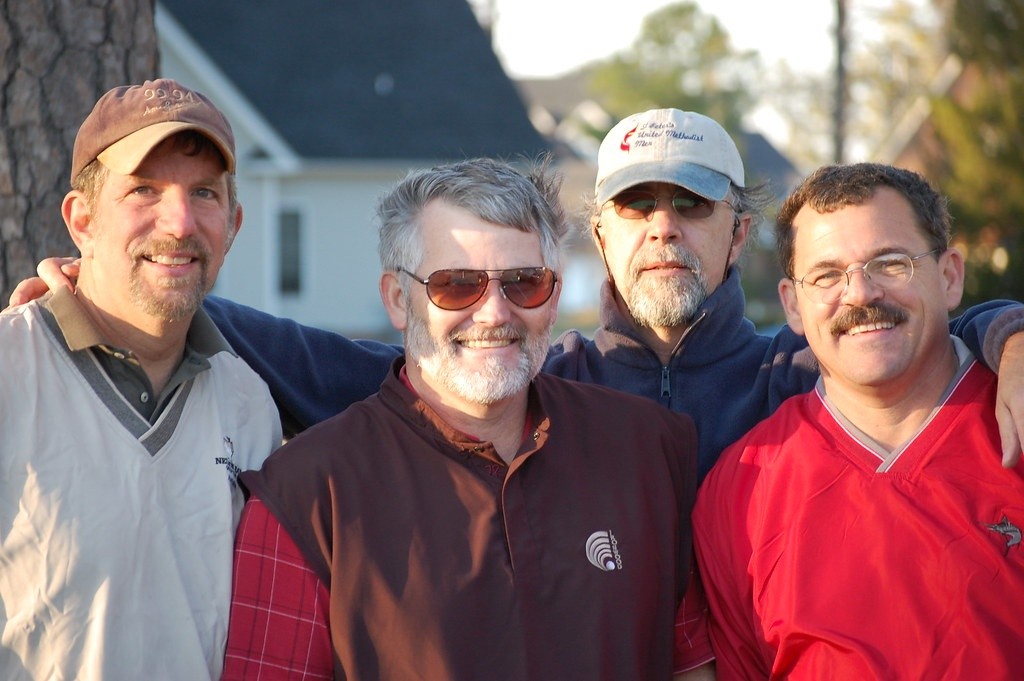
[
  {"xmin": 70, "ymin": 77, "xmax": 235, "ymax": 189},
  {"xmin": 593, "ymin": 108, "xmax": 745, "ymax": 208}
]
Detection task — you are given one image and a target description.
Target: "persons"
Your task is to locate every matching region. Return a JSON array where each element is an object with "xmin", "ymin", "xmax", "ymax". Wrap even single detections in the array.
[
  {"xmin": 0, "ymin": 108, "xmax": 1023, "ymax": 681},
  {"xmin": 0, "ymin": 78, "xmax": 283, "ymax": 681},
  {"xmin": 222, "ymin": 157, "xmax": 697, "ymax": 681},
  {"xmin": 679, "ymin": 160, "xmax": 1024, "ymax": 681}
]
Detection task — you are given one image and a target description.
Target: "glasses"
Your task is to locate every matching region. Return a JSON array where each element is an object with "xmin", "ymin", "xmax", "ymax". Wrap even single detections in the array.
[
  {"xmin": 396, "ymin": 267, "xmax": 558, "ymax": 311},
  {"xmin": 610, "ymin": 191, "xmax": 715, "ymax": 220},
  {"xmin": 790, "ymin": 249, "xmax": 941, "ymax": 304}
]
